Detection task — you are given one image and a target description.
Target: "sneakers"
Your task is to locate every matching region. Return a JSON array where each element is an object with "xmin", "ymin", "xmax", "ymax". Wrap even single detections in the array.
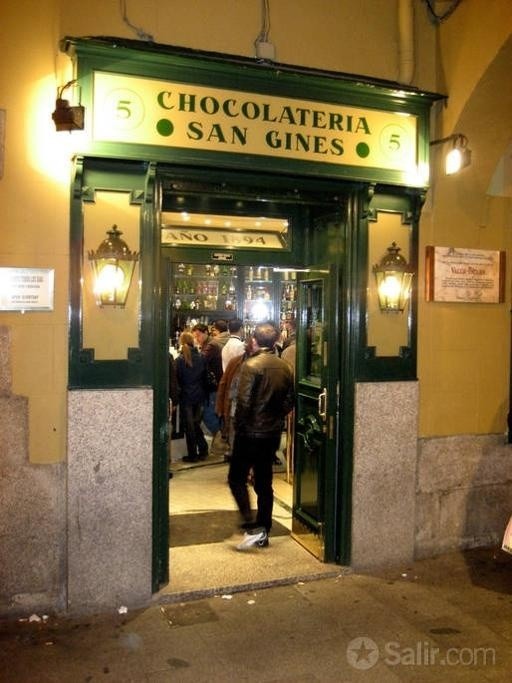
[
  {"xmin": 235, "ymin": 527, "xmax": 270, "ymax": 551},
  {"xmin": 181, "ymin": 450, "xmax": 208, "ymax": 463}
]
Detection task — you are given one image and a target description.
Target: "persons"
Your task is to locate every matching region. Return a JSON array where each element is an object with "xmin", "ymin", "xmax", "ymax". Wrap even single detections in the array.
[
  {"xmin": 223, "ymin": 321, "xmax": 294, "ymax": 549},
  {"xmin": 167, "ymin": 315, "xmax": 295, "ymax": 477}
]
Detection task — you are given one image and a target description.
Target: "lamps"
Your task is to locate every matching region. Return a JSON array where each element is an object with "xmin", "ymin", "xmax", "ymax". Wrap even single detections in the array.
[
  {"xmin": 372, "ymin": 241, "xmax": 415, "ymax": 314},
  {"xmin": 52, "ymin": 79, "xmax": 85, "ymax": 134},
  {"xmin": 429, "ymin": 131, "xmax": 472, "ymax": 175},
  {"xmin": 88, "ymin": 223, "xmax": 140, "ymax": 308}
]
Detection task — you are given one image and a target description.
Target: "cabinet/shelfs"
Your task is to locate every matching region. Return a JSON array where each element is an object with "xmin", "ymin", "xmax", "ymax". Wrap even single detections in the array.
[{"xmin": 169, "ymin": 262, "xmax": 298, "ymax": 347}]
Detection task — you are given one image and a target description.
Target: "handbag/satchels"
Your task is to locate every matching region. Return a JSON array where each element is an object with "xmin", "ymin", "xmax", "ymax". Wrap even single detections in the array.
[
  {"xmin": 210, "ymin": 428, "xmax": 231, "ymax": 460},
  {"xmin": 206, "ymin": 372, "xmax": 218, "ymax": 392}
]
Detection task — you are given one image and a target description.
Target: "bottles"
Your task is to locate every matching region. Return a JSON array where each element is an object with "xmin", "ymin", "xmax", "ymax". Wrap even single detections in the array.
[
  {"xmin": 174, "ymin": 316, "xmax": 210, "ymax": 342},
  {"xmin": 173, "ymin": 263, "xmax": 236, "ymax": 311},
  {"xmin": 281, "ymin": 311, "xmax": 294, "ymax": 321},
  {"xmin": 246, "ymin": 284, "xmax": 297, "ymax": 301},
  {"xmin": 248, "ymin": 267, "xmax": 297, "ymax": 281}
]
